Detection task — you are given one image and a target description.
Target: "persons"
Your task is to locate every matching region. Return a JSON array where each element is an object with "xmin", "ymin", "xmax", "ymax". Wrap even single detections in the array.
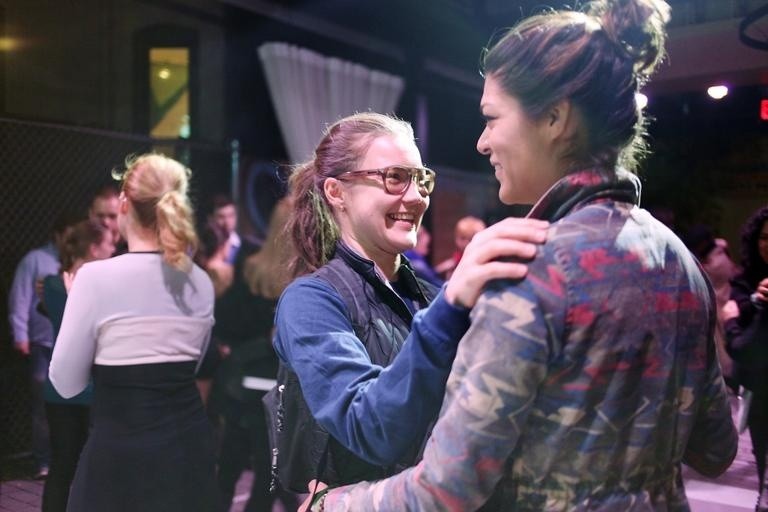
[
  {"xmin": 290, "ymin": 0, "xmax": 736, "ymax": 511},
  {"xmin": 404, "ymin": 224, "xmax": 443, "ymax": 288},
  {"xmin": 191, "ymin": 193, "xmax": 279, "ymax": 464},
  {"xmin": 46, "ymin": 152, "xmax": 215, "ymax": 512},
  {"xmin": 654, "ymin": 203, "xmax": 768, "ymax": 477},
  {"xmin": 6, "ymin": 182, "xmax": 128, "ymax": 512},
  {"xmin": 437, "ymin": 216, "xmax": 485, "ymax": 274},
  {"xmin": 245, "ymin": 114, "xmax": 551, "ymax": 512}
]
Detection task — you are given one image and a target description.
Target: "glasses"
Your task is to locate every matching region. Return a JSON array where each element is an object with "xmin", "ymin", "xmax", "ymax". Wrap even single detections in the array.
[{"xmin": 343, "ymin": 165, "xmax": 436, "ymax": 195}]
